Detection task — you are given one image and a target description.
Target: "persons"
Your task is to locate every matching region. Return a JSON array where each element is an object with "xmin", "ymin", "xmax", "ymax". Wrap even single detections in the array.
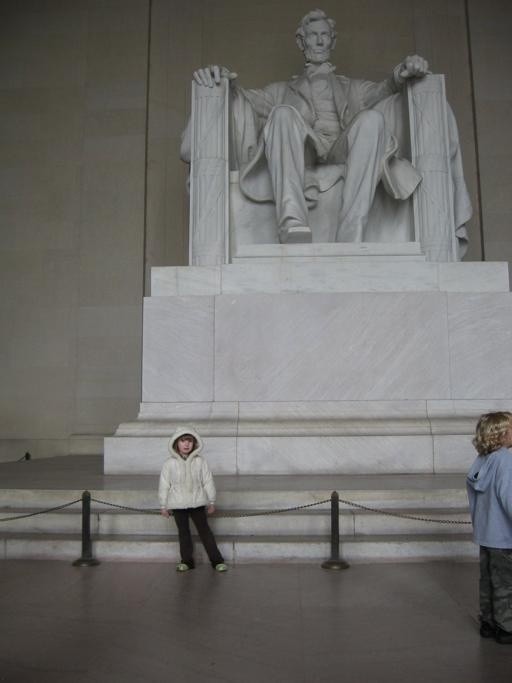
[
  {"xmin": 188, "ymin": 7, "xmax": 436, "ymax": 245},
  {"xmin": 464, "ymin": 408, "xmax": 512, "ymax": 645},
  {"xmin": 155, "ymin": 423, "xmax": 231, "ymax": 573}
]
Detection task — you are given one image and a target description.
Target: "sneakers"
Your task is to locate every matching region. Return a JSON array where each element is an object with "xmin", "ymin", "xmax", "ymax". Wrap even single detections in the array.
[
  {"xmin": 480, "ymin": 621, "xmax": 512, "ymax": 645},
  {"xmin": 215, "ymin": 563, "xmax": 228, "ymax": 572},
  {"xmin": 177, "ymin": 563, "xmax": 190, "ymax": 571}
]
